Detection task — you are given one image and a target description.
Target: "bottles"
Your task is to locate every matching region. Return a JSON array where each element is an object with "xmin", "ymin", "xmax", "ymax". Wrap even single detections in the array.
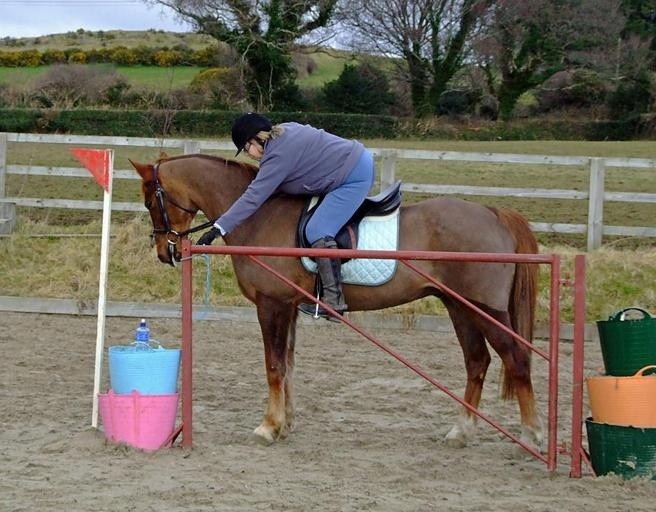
[{"xmin": 135, "ymin": 319, "xmax": 150, "ymax": 352}]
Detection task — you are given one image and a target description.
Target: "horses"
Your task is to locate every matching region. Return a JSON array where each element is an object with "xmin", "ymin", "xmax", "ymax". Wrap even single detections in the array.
[{"xmin": 127, "ymin": 149, "xmax": 542, "ymax": 462}]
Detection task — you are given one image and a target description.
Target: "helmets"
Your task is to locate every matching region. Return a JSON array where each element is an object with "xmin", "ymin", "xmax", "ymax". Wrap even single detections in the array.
[{"xmin": 231, "ymin": 112, "xmax": 271, "ymax": 158}]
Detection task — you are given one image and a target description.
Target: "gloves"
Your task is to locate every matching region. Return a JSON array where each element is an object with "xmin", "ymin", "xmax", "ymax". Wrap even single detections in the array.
[{"xmin": 196, "ymin": 228, "xmax": 219, "ymax": 244}]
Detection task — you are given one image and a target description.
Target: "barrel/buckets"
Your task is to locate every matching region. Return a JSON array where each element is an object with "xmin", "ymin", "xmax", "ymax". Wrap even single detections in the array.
[
  {"xmin": 110, "ymin": 346, "xmax": 181, "ymax": 394},
  {"xmin": 96, "ymin": 393, "xmax": 179, "ymax": 451},
  {"xmin": 586, "ymin": 375, "xmax": 656, "ymax": 428},
  {"xmin": 579, "ymin": 419, "xmax": 656, "ymax": 481},
  {"xmin": 597, "ymin": 307, "xmax": 656, "ymax": 375}
]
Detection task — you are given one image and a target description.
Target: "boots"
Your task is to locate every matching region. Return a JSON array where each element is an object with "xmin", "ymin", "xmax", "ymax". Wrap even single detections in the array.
[{"xmin": 298, "ymin": 236, "xmax": 349, "ymax": 317}]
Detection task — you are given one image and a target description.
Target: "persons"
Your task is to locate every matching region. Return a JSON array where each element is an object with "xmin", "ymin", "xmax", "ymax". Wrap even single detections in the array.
[{"xmin": 197, "ymin": 112, "xmax": 374, "ymax": 325}]
